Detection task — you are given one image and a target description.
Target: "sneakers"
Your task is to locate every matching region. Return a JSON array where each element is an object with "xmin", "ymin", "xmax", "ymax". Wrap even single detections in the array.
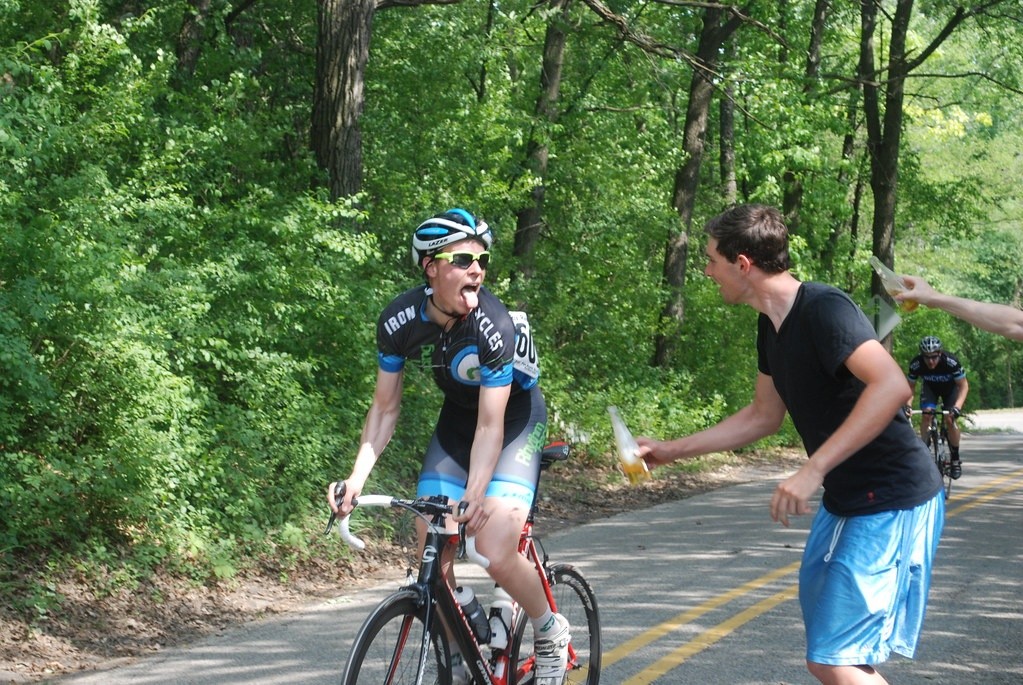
[
  {"xmin": 534, "ymin": 613, "xmax": 572, "ymax": 685},
  {"xmin": 433, "ymin": 653, "xmax": 473, "ymax": 685}
]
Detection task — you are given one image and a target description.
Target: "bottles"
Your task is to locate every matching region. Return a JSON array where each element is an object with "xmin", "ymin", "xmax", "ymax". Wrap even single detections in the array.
[
  {"xmin": 938, "ymin": 439, "xmax": 952, "ymax": 464},
  {"xmin": 868, "ymin": 256, "xmax": 919, "ymax": 313},
  {"xmin": 453, "ymin": 586, "xmax": 492, "ymax": 650},
  {"xmin": 606, "ymin": 405, "xmax": 653, "ymax": 489},
  {"xmin": 487, "ymin": 580, "xmax": 512, "ymax": 650}
]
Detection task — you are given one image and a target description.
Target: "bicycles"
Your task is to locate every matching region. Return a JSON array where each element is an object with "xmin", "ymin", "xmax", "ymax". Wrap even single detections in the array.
[
  {"xmin": 321, "ymin": 481, "xmax": 601, "ymax": 685},
  {"xmin": 905, "ymin": 407, "xmax": 961, "ymax": 499}
]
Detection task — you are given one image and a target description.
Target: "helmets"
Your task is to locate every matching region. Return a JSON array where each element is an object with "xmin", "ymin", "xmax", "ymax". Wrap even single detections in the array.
[
  {"xmin": 919, "ymin": 337, "xmax": 942, "ymax": 353},
  {"xmin": 412, "ymin": 208, "xmax": 494, "ymax": 270}
]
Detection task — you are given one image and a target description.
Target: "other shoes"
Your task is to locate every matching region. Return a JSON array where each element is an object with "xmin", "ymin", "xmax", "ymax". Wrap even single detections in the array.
[{"xmin": 951, "ymin": 459, "xmax": 962, "ymax": 480}]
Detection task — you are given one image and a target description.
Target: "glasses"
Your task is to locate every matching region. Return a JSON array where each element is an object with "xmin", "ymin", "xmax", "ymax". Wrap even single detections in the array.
[
  {"xmin": 922, "ymin": 353, "xmax": 940, "ymax": 360},
  {"xmin": 434, "ymin": 250, "xmax": 493, "ymax": 270}
]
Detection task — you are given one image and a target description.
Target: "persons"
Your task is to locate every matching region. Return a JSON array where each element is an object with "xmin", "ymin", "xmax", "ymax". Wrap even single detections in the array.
[
  {"xmin": 895, "ymin": 274, "xmax": 1023, "ymax": 341},
  {"xmin": 907, "ymin": 336, "xmax": 970, "ymax": 480},
  {"xmin": 638, "ymin": 203, "xmax": 944, "ymax": 685},
  {"xmin": 327, "ymin": 209, "xmax": 571, "ymax": 685}
]
